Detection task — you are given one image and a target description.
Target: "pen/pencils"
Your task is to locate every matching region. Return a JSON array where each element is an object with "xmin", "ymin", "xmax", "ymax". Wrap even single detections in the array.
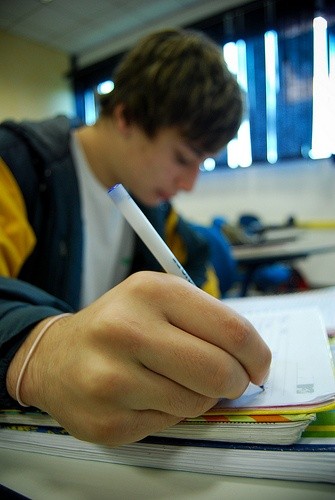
[{"xmin": 107, "ymin": 183, "xmax": 271, "ymax": 391}]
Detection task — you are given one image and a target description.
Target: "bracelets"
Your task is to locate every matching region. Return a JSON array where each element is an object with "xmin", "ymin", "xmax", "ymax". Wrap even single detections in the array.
[{"xmin": 16, "ymin": 311, "xmax": 76, "ymax": 407}]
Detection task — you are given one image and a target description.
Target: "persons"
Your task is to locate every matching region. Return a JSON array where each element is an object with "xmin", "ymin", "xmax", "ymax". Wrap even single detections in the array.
[{"xmin": 0, "ymin": 26, "xmax": 272, "ymax": 451}]
